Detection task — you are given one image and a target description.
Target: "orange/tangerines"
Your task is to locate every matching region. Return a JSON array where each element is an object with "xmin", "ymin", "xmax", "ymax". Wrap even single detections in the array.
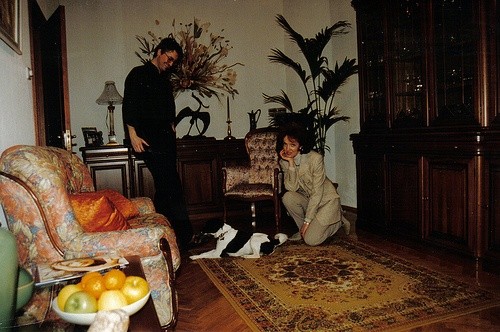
[{"xmin": 82, "ymin": 269, "xmax": 125, "ymax": 300}]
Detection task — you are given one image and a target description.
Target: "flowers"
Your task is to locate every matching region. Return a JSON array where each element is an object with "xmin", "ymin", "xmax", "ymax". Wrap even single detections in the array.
[{"xmin": 132, "ymin": 16, "xmax": 245, "ymax": 102}]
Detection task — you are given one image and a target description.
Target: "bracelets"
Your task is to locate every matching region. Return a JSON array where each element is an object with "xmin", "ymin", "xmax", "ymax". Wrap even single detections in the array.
[{"xmin": 303, "ymin": 221, "xmax": 310, "ymax": 225}]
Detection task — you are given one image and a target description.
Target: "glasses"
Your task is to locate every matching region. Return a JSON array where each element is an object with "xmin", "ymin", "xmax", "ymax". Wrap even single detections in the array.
[{"xmin": 161, "ymin": 49, "xmax": 177, "ymax": 64}]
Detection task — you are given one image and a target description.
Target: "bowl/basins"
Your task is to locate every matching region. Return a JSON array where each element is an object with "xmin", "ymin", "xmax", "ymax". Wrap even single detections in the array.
[{"xmin": 52, "ymin": 287, "xmax": 152, "ymax": 325}]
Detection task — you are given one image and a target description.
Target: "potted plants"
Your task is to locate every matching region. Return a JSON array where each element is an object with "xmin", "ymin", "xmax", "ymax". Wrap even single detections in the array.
[{"xmin": 261, "ymin": 14, "xmax": 359, "ymax": 191}]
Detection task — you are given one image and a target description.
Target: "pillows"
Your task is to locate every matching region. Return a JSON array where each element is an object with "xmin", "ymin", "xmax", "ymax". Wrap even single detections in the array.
[
  {"xmin": 68, "ymin": 191, "xmax": 132, "ymax": 232},
  {"xmin": 96, "ymin": 187, "xmax": 139, "ymax": 221}
]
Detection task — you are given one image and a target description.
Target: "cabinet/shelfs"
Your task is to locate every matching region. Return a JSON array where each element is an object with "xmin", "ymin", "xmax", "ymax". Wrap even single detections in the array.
[
  {"xmin": 79, "ymin": 142, "xmax": 248, "ymax": 228},
  {"xmin": 349, "ymin": 0, "xmax": 500, "ymax": 264}
]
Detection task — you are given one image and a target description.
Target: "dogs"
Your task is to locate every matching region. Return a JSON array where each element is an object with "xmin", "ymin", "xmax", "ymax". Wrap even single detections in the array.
[{"xmin": 186, "ymin": 217, "xmax": 291, "ymax": 261}]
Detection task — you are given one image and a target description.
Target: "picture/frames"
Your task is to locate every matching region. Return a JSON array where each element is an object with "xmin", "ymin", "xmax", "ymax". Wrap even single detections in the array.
[
  {"xmin": 81, "ymin": 126, "xmax": 98, "ymax": 147},
  {"xmin": 0, "ymin": 0, "xmax": 24, "ymax": 54}
]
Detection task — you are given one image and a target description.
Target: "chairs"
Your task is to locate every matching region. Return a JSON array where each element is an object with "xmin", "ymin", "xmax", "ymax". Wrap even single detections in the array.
[{"xmin": 220, "ymin": 128, "xmax": 291, "ymax": 237}]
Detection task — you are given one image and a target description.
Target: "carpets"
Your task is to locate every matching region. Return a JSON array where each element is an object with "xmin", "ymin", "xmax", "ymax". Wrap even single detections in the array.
[{"xmin": 190, "ymin": 226, "xmax": 499, "ymax": 332}]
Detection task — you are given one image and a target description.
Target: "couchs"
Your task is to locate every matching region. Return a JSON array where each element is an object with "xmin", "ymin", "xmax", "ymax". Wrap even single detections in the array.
[{"xmin": 1, "ymin": 143, "xmax": 182, "ymax": 332}]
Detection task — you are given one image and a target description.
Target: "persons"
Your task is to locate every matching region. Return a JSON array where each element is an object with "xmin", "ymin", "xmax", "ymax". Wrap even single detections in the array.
[
  {"xmin": 122, "ymin": 38, "xmax": 208, "ymax": 247},
  {"xmin": 276, "ymin": 121, "xmax": 350, "ymax": 246}
]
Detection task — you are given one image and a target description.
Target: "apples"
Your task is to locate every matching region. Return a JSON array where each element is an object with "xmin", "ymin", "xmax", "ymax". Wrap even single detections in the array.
[{"xmin": 57, "ymin": 276, "xmax": 148, "ymax": 314}]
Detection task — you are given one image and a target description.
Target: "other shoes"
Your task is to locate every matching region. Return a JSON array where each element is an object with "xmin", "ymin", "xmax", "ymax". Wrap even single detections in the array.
[
  {"xmin": 287, "ymin": 232, "xmax": 302, "ymax": 241},
  {"xmin": 187, "ymin": 232, "xmax": 210, "ymax": 249},
  {"xmin": 340, "ymin": 215, "xmax": 350, "ymax": 234}
]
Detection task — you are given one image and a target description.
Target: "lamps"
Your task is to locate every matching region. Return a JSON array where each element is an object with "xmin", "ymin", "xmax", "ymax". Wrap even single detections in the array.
[{"xmin": 96, "ymin": 81, "xmax": 124, "ymax": 148}]
